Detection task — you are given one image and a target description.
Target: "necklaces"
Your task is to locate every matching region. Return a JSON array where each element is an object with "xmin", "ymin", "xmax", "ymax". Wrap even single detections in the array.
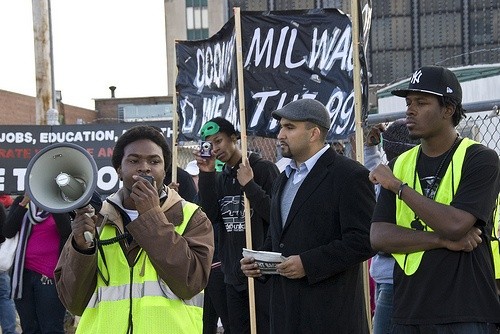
[{"xmin": 411, "ymin": 133, "xmax": 460, "ymax": 228}]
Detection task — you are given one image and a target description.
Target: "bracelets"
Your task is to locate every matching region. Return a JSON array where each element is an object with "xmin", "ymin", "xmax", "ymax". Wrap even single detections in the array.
[
  {"xmin": 19, "ymin": 202, "xmax": 26, "ymax": 207},
  {"xmin": 398, "ymin": 182, "xmax": 407, "ymax": 199}
]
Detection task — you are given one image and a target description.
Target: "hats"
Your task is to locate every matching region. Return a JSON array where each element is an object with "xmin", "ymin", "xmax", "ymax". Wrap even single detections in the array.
[
  {"xmin": 271, "ymin": 98, "xmax": 330, "ymax": 130},
  {"xmin": 391, "ymin": 66, "xmax": 462, "ymax": 102},
  {"xmin": 382, "ymin": 118, "xmax": 421, "ymax": 154},
  {"xmin": 112, "ymin": 125, "xmax": 171, "ymax": 173},
  {"xmin": 201, "ymin": 117, "xmax": 241, "ymax": 140}
]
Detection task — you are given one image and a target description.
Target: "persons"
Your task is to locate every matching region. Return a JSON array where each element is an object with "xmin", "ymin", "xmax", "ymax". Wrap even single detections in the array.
[
  {"xmin": 163, "ymin": 159, "xmax": 230, "ymax": 334},
  {"xmin": 240, "ymin": 99, "xmax": 377, "ymax": 334},
  {"xmin": 364, "ymin": 119, "xmax": 417, "ymax": 334},
  {"xmin": 369, "ymin": 66, "xmax": 500, "ymax": 334},
  {"xmin": 7, "ymin": 191, "xmax": 77, "ymax": 334},
  {"xmin": 192, "ymin": 117, "xmax": 280, "ymax": 334},
  {"xmin": 54, "ymin": 126, "xmax": 215, "ymax": 334},
  {"xmin": 0, "ymin": 194, "xmax": 24, "ymax": 334}
]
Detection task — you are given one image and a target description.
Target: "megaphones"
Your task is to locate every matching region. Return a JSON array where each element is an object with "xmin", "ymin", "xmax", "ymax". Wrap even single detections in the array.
[{"xmin": 24, "ymin": 142, "xmax": 102, "ymax": 243}]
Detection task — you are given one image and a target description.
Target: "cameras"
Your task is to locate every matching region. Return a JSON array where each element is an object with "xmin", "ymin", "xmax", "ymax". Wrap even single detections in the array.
[{"xmin": 200, "ymin": 141, "xmax": 212, "ymax": 157}]
